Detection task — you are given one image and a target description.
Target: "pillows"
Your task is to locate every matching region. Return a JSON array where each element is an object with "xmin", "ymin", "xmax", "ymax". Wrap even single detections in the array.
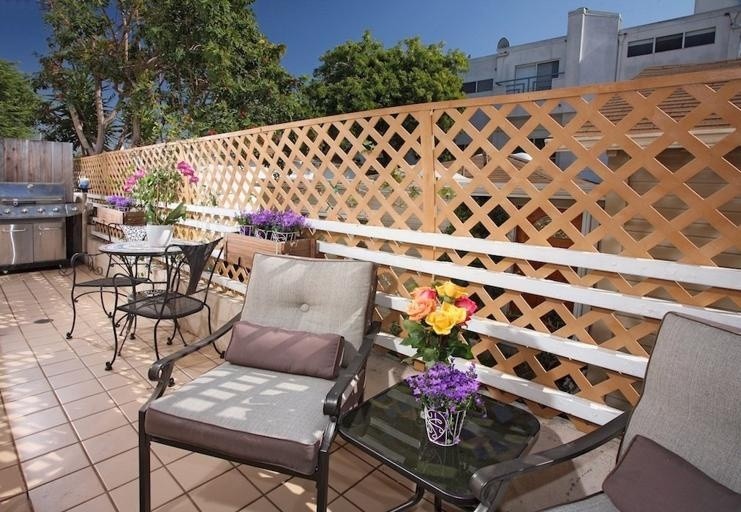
[
  {"xmin": 225, "ymin": 321, "xmax": 345, "ymax": 380},
  {"xmin": 600, "ymin": 434, "xmax": 741, "ymax": 512}
]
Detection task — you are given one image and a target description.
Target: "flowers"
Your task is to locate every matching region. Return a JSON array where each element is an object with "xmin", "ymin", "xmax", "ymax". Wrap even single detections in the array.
[
  {"xmin": 405, "ymin": 364, "xmax": 488, "ymax": 413},
  {"xmin": 122, "ymin": 164, "xmax": 198, "ymax": 225},
  {"xmin": 234, "ymin": 210, "xmax": 252, "ymax": 225},
  {"xmin": 252, "ymin": 210, "xmax": 274, "ymax": 228},
  {"xmin": 274, "ymin": 210, "xmax": 304, "ymax": 232}
]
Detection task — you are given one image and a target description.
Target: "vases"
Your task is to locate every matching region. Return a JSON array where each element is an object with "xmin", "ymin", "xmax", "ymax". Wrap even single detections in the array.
[
  {"xmin": 239, "ymin": 226, "xmax": 252, "ymax": 236},
  {"xmin": 272, "ymin": 232, "xmax": 296, "ymax": 241},
  {"xmin": 145, "ymin": 225, "xmax": 171, "ymax": 248},
  {"xmin": 255, "ymin": 229, "xmax": 273, "ymax": 239}
]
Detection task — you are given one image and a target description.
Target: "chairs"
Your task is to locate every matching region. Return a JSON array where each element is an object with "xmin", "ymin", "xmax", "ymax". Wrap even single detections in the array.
[
  {"xmin": 105, "ymin": 239, "xmax": 224, "ymax": 387},
  {"xmin": 65, "ymin": 223, "xmax": 154, "ymax": 339},
  {"xmin": 468, "ymin": 309, "xmax": 741, "ymax": 512},
  {"xmin": 138, "ymin": 252, "xmax": 380, "ymax": 512}
]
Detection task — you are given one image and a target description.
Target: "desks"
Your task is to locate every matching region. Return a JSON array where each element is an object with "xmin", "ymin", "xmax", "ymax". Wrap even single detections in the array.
[
  {"xmin": 339, "ymin": 376, "xmax": 541, "ymax": 512},
  {"xmin": 97, "ymin": 240, "xmax": 186, "ymax": 354}
]
